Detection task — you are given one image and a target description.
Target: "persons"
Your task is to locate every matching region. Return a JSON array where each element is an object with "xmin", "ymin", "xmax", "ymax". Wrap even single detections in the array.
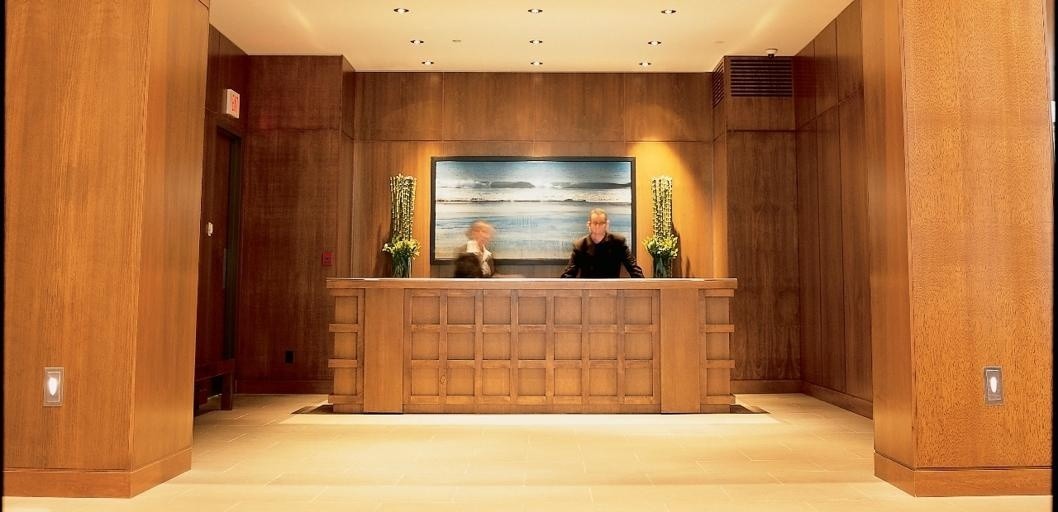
[
  {"xmin": 451, "ymin": 218, "xmax": 522, "ymax": 278},
  {"xmin": 559, "ymin": 208, "xmax": 645, "ymax": 277}
]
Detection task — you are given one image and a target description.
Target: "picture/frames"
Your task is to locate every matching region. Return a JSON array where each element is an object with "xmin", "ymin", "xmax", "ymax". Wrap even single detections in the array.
[{"xmin": 429, "ymin": 156, "xmax": 636, "ymax": 265}]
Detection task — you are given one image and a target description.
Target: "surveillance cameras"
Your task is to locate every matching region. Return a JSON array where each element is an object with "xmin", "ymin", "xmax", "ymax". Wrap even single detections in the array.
[{"xmin": 766, "ymin": 50, "xmax": 777, "ymax": 59}]
